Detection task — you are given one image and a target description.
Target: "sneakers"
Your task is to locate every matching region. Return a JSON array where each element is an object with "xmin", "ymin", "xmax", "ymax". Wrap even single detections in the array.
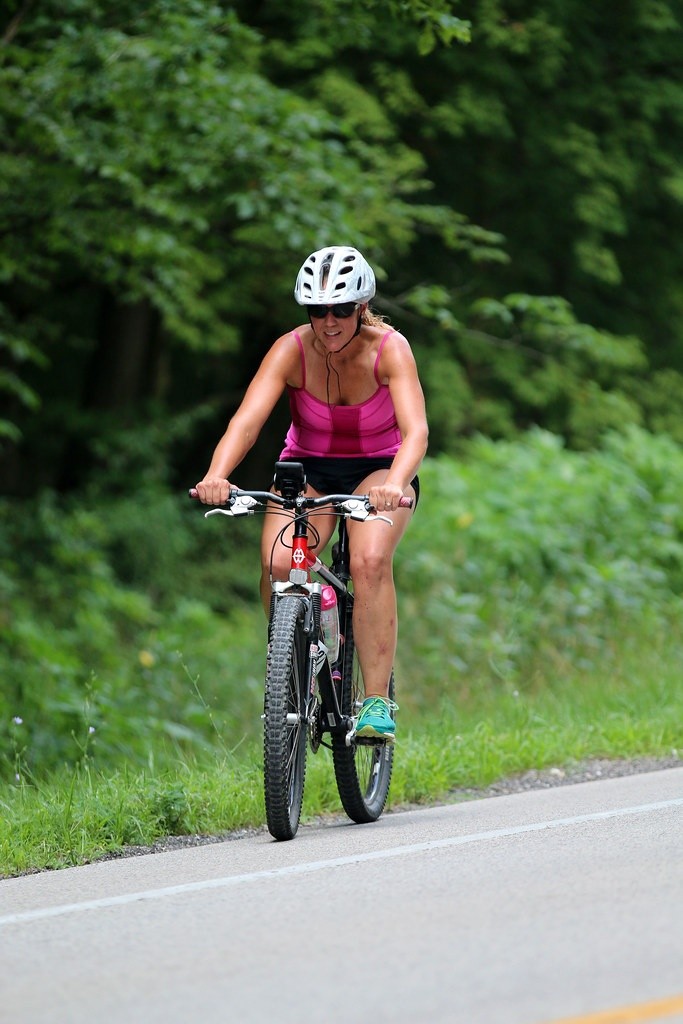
[{"xmin": 356, "ymin": 696, "xmax": 399, "ymax": 739}]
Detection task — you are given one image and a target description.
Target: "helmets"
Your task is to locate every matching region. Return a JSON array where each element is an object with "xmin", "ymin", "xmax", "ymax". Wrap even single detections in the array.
[{"xmin": 294, "ymin": 245, "xmax": 376, "ymax": 304}]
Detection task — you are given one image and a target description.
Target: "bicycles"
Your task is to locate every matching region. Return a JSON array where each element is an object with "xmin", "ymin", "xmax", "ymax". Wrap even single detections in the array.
[{"xmin": 187, "ymin": 459, "xmax": 414, "ymax": 842}]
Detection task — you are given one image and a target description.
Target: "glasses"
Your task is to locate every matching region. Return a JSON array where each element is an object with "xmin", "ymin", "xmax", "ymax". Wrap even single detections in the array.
[
  {"xmin": 355, "ymin": 319, "xmax": 356, "ymax": 322},
  {"xmin": 307, "ymin": 302, "xmax": 356, "ymax": 324}
]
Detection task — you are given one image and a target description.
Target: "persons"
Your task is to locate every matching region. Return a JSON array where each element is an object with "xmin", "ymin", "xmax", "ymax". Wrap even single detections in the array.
[{"xmin": 196, "ymin": 246, "xmax": 429, "ymax": 745}]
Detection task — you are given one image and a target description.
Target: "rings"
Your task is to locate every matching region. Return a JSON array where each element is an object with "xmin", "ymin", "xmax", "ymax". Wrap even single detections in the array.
[{"xmin": 385, "ymin": 503, "xmax": 391, "ymax": 506}]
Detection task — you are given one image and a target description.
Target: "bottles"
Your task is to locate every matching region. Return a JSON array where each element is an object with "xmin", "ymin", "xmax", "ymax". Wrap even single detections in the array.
[{"xmin": 320, "ymin": 584, "xmax": 339, "ymax": 664}]
